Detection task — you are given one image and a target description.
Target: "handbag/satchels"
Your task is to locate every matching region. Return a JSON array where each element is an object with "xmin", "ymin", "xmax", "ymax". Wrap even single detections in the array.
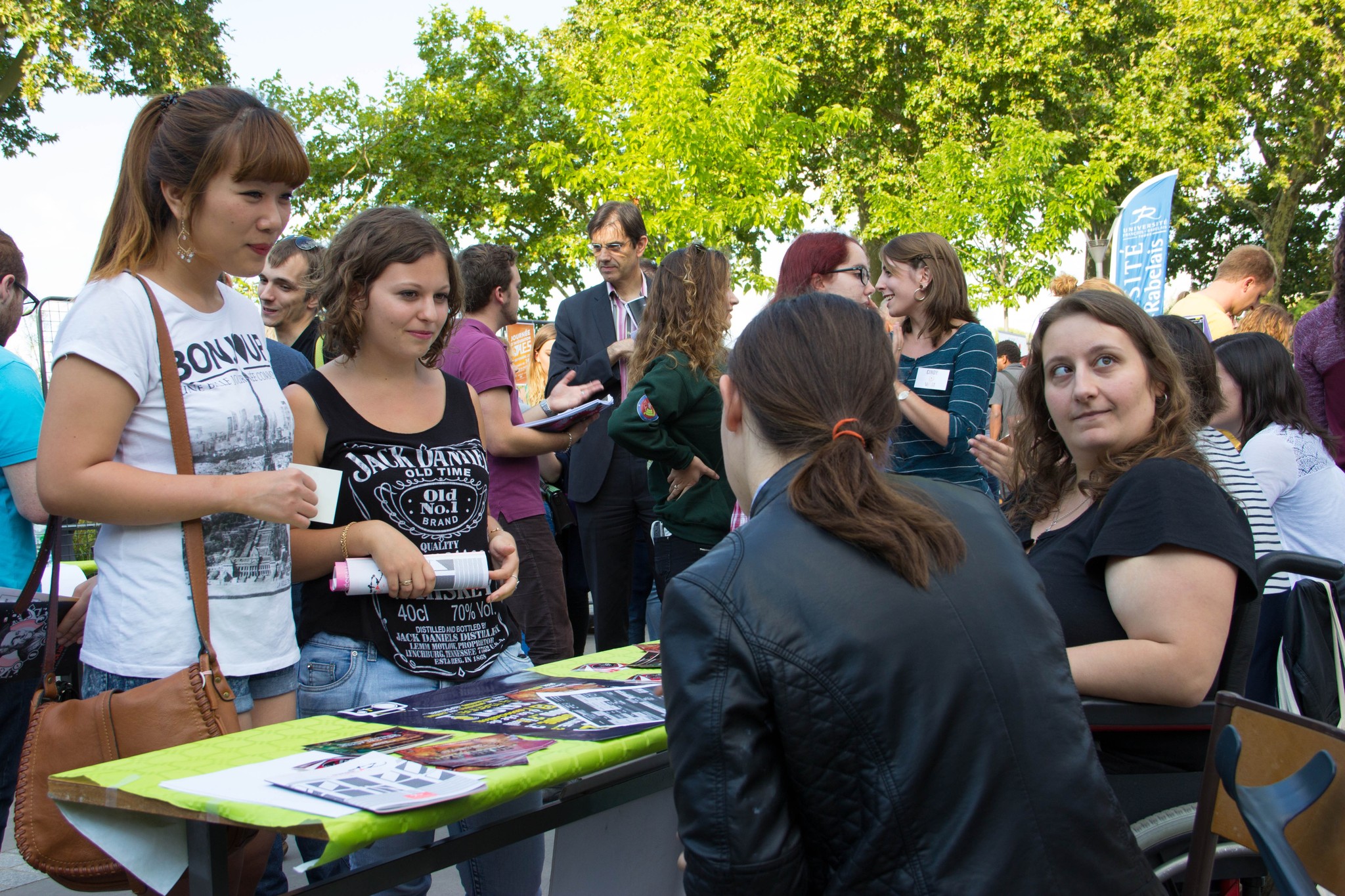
[{"xmin": 14, "ymin": 652, "xmax": 261, "ymax": 891}]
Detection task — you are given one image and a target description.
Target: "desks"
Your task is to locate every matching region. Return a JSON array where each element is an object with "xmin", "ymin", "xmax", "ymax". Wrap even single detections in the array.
[{"xmin": 47, "ymin": 632, "xmax": 675, "ymax": 896}]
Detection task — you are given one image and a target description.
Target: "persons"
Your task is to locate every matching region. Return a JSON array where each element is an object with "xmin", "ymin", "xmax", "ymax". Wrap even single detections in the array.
[
  {"xmin": 39, "ymin": 87, "xmax": 318, "ymax": 895},
  {"xmin": 283, "ymin": 208, "xmax": 548, "ymax": 895},
  {"xmin": 0, "ymin": 226, "xmax": 69, "ymax": 856},
  {"xmin": 57, "ymin": 335, "xmax": 316, "ymax": 650},
  {"xmin": 998, "ymin": 292, "xmax": 1265, "ymax": 860},
  {"xmin": 252, "ymin": 201, "xmax": 1345, "ymax": 701},
  {"xmin": 659, "ymin": 299, "xmax": 1177, "ymax": 896}
]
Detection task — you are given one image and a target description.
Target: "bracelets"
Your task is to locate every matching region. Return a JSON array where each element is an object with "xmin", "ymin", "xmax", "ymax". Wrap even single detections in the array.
[
  {"xmin": 897, "ymin": 387, "xmax": 911, "ymax": 401},
  {"xmin": 561, "ymin": 430, "xmax": 573, "ymax": 454},
  {"xmin": 487, "ymin": 528, "xmax": 503, "ymax": 535},
  {"xmin": 540, "ymin": 400, "xmax": 555, "ymax": 417},
  {"xmin": 340, "ymin": 517, "xmax": 356, "ymax": 562}
]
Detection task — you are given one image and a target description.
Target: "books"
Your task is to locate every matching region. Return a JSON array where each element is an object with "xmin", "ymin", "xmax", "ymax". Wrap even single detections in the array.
[{"xmin": 264, "ymin": 750, "xmax": 489, "ymax": 814}]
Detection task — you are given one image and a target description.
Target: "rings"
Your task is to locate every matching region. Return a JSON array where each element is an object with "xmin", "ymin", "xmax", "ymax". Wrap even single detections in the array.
[
  {"xmin": 674, "ymin": 484, "xmax": 681, "ymax": 493},
  {"xmin": 511, "ymin": 574, "xmax": 519, "ymax": 591},
  {"xmin": 581, "ymin": 391, "xmax": 585, "ymax": 395},
  {"xmin": 401, "ymin": 580, "xmax": 412, "ymax": 587}
]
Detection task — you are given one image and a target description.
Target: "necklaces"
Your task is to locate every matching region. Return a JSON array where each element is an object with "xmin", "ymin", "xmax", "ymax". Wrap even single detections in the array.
[{"xmin": 1045, "ymin": 488, "xmax": 1097, "ymax": 532}]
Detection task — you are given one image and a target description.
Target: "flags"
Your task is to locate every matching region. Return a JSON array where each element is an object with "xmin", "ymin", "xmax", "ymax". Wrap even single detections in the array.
[{"xmin": 1109, "ymin": 167, "xmax": 1180, "ymax": 316}]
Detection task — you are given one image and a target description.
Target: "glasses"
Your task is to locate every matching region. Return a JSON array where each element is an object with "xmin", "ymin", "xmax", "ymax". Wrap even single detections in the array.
[
  {"xmin": 822, "ymin": 266, "xmax": 870, "ymax": 286},
  {"xmin": 586, "ymin": 239, "xmax": 632, "ymax": 256},
  {"xmin": 276, "ymin": 235, "xmax": 318, "ymax": 250},
  {"xmin": 13, "ymin": 282, "xmax": 40, "ymax": 317},
  {"xmin": 693, "ymin": 244, "xmax": 707, "ymax": 253}
]
